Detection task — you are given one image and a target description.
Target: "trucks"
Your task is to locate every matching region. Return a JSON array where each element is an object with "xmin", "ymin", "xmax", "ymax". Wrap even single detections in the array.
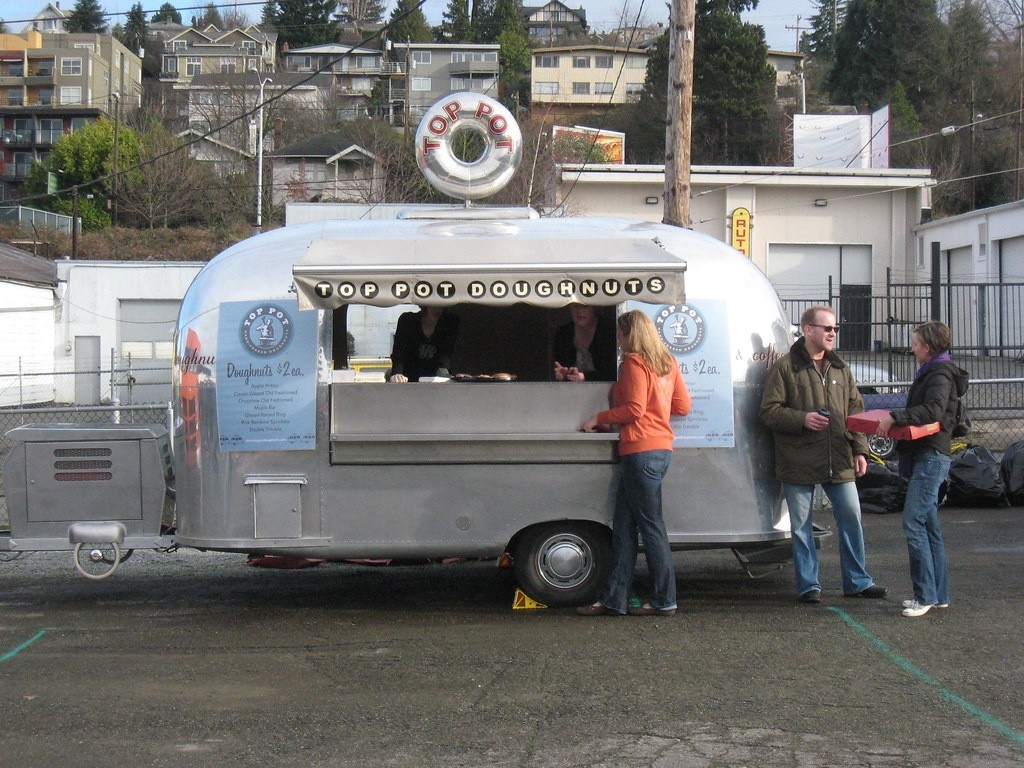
[{"xmin": 0, "ymin": 92, "xmax": 834, "ymax": 612}]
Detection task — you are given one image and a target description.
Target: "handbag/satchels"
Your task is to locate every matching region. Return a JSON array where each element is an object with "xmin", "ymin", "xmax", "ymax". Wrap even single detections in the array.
[{"xmin": 952, "ymin": 400, "xmax": 972, "ymax": 437}]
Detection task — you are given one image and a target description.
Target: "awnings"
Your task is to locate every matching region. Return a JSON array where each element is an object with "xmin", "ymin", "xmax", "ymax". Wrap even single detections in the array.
[{"xmin": 292, "ymin": 239, "xmax": 688, "ymax": 311}]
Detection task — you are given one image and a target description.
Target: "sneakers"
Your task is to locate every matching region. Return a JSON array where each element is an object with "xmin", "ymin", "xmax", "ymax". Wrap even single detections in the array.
[
  {"xmin": 627, "ymin": 605, "xmax": 676, "ymax": 616},
  {"xmin": 577, "ymin": 604, "xmax": 625, "ymax": 616},
  {"xmin": 902, "ymin": 599, "xmax": 948, "ymax": 617}
]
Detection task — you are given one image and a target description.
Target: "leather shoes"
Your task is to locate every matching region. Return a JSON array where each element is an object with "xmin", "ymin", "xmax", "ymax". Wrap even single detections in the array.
[
  {"xmin": 801, "ymin": 590, "xmax": 821, "ymax": 602},
  {"xmin": 844, "ymin": 585, "xmax": 890, "ymax": 598}
]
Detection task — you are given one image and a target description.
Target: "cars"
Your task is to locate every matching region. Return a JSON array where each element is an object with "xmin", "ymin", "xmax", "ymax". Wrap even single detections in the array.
[{"xmin": 854, "ymin": 387, "xmax": 909, "ymax": 461}]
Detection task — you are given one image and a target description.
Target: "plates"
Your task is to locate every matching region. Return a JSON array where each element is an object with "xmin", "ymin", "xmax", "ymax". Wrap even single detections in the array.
[{"xmin": 451, "ymin": 376, "xmax": 518, "ymax": 383}]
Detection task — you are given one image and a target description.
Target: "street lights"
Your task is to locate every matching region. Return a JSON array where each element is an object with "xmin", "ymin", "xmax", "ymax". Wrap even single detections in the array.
[{"xmin": 252, "ymin": 67, "xmax": 272, "ymax": 228}]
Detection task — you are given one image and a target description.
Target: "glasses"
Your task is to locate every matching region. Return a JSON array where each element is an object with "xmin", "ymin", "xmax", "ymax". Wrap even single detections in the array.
[{"xmin": 809, "ymin": 324, "xmax": 840, "ymax": 333}]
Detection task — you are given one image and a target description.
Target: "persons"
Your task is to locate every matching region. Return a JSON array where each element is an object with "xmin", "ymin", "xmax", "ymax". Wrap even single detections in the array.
[
  {"xmin": 875, "ymin": 321, "xmax": 969, "ymax": 617},
  {"xmin": 390, "ymin": 305, "xmax": 460, "ymax": 383},
  {"xmin": 577, "ymin": 310, "xmax": 691, "ymax": 617},
  {"xmin": 552, "ymin": 303, "xmax": 616, "ymax": 382},
  {"xmin": 757, "ymin": 306, "xmax": 890, "ymax": 601}
]
